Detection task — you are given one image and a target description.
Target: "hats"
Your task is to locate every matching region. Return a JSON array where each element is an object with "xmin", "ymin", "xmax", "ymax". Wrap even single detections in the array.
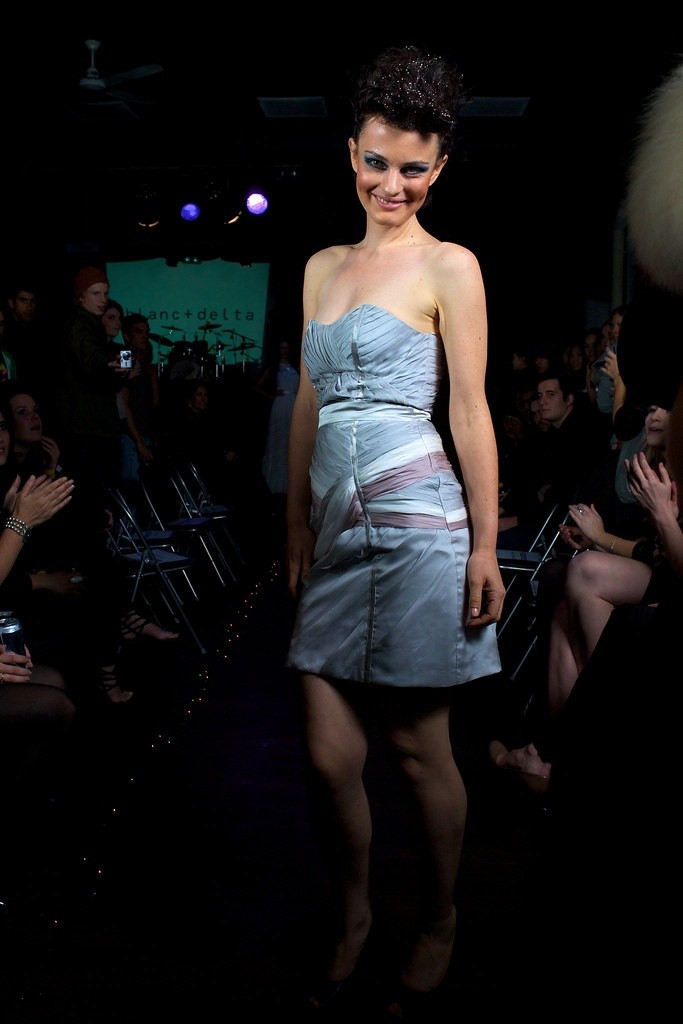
[{"xmin": 75, "ymin": 266, "xmax": 111, "ymax": 299}]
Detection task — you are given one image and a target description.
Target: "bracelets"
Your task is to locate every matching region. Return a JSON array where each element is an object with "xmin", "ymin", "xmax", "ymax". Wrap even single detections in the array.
[{"xmin": 610, "ymin": 537, "xmax": 616, "ymax": 552}]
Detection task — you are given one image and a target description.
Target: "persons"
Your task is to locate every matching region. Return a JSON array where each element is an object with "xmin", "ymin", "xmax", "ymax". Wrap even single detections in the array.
[
  {"xmin": 0, "ymin": 268, "xmax": 297, "ymax": 781},
  {"xmin": 480, "ymin": 68, "xmax": 683, "ymax": 794},
  {"xmin": 286, "ymin": 43, "xmax": 508, "ymax": 1020}
]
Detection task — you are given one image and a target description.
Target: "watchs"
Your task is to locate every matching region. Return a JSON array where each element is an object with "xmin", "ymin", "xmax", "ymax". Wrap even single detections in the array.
[{"xmin": 5, "ymin": 517, "xmax": 33, "ymax": 540}]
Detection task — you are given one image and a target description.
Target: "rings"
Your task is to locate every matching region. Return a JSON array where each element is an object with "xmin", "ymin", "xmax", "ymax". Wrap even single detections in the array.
[{"xmin": 579, "ymin": 510, "xmax": 583, "ymax": 513}]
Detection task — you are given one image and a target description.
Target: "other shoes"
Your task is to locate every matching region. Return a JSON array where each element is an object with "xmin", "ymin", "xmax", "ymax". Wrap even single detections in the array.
[
  {"xmin": 382, "ymin": 970, "xmax": 448, "ymax": 1024},
  {"xmin": 305, "ymin": 910, "xmax": 384, "ymax": 1011},
  {"xmin": 488, "ymin": 739, "xmax": 550, "ymax": 795}
]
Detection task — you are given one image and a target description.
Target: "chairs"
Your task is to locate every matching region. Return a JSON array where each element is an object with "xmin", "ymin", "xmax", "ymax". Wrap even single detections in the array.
[
  {"xmin": 490, "ymin": 481, "xmax": 587, "ymax": 719},
  {"xmin": 101, "ymin": 459, "xmax": 251, "ymax": 631}
]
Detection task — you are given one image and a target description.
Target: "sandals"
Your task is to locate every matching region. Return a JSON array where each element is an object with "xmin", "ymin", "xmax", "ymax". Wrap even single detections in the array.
[
  {"xmin": 117, "ymin": 611, "xmax": 178, "ymax": 648},
  {"xmin": 90, "ymin": 669, "xmax": 138, "ymax": 708}
]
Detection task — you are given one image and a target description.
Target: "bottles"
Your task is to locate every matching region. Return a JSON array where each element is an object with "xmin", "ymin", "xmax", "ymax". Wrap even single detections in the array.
[
  {"xmin": 498, "ymin": 480, "xmax": 505, "ymax": 494},
  {"xmin": 0, "ymin": 615, "xmax": 29, "ymax": 674}
]
